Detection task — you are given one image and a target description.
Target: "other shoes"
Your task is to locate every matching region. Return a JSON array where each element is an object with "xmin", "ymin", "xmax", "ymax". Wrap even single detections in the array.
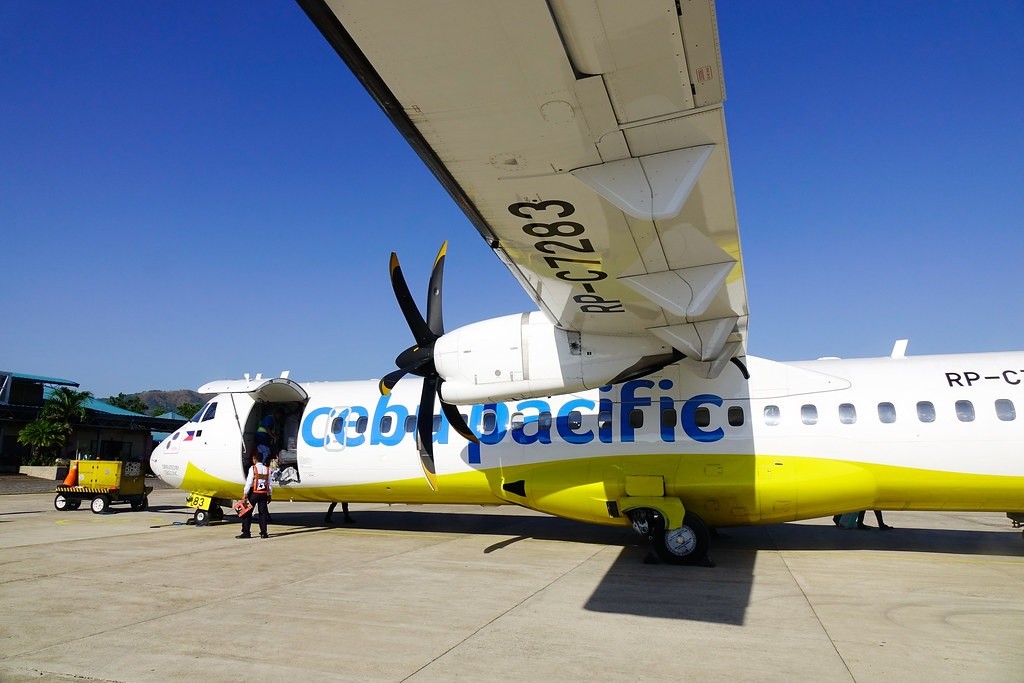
[
  {"xmin": 880, "ymin": 526, "xmax": 893, "ymax": 529},
  {"xmin": 325, "ymin": 518, "xmax": 335, "ymax": 523},
  {"xmin": 261, "ymin": 534, "xmax": 269, "ymax": 538},
  {"xmin": 859, "ymin": 523, "xmax": 871, "ymax": 530},
  {"xmin": 346, "ymin": 519, "xmax": 355, "ymax": 523},
  {"xmin": 236, "ymin": 532, "xmax": 250, "ymax": 538}
]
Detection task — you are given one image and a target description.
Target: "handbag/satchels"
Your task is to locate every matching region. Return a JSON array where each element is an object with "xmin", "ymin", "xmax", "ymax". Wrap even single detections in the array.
[{"xmin": 278, "ymin": 448, "xmax": 297, "ymax": 466}]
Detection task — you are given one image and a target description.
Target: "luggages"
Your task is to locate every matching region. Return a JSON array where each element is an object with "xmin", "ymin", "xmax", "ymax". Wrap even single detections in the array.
[{"xmin": 839, "ymin": 512, "xmax": 859, "ymax": 529}]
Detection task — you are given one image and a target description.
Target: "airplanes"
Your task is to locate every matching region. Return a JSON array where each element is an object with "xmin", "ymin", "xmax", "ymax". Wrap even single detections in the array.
[{"xmin": 148, "ymin": 0, "xmax": 1024, "ymax": 568}]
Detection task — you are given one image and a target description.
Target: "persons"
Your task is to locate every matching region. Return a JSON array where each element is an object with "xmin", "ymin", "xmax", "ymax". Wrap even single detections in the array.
[
  {"xmin": 325, "ymin": 502, "xmax": 357, "ymax": 524},
  {"xmin": 857, "ymin": 510, "xmax": 894, "ymax": 530},
  {"xmin": 255, "ymin": 408, "xmax": 285, "ymax": 467},
  {"xmin": 82, "ymin": 451, "xmax": 89, "ymax": 460},
  {"xmin": 235, "ymin": 453, "xmax": 272, "ymax": 539}
]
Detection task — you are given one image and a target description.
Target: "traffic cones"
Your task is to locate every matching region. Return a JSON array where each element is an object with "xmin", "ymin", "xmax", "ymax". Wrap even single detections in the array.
[{"xmin": 57, "ymin": 462, "xmax": 80, "ymax": 489}]
